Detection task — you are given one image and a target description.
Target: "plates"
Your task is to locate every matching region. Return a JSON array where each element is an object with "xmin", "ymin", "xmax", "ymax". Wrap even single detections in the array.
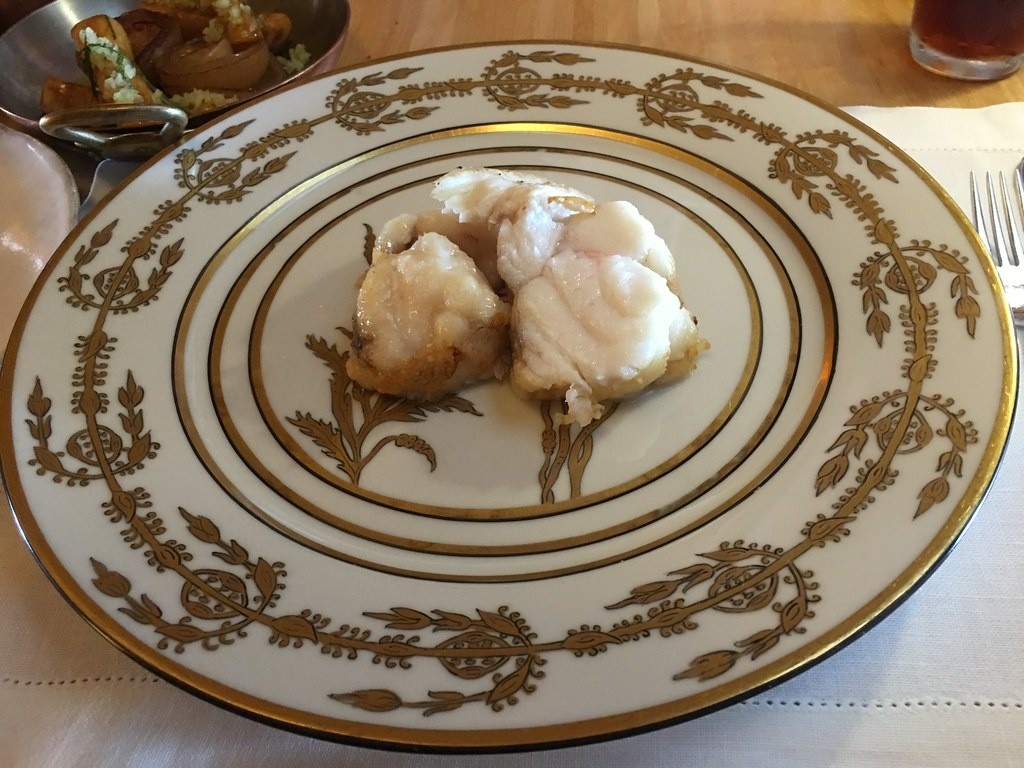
[
  {"xmin": 0, "ymin": 124, "xmax": 82, "ymax": 410},
  {"xmin": 0, "ymin": 41, "xmax": 1019, "ymax": 755}
]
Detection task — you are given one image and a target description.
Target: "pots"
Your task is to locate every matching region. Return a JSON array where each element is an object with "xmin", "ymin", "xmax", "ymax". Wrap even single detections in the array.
[{"xmin": 0, "ymin": 0, "xmax": 351, "ymax": 158}]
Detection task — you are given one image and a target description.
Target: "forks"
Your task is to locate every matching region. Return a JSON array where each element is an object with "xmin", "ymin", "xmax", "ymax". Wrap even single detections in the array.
[{"xmin": 969, "ymin": 168, "xmax": 1024, "ymax": 336}]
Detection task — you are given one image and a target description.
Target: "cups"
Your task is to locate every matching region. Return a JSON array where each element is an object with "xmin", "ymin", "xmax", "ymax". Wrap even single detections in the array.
[{"xmin": 907, "ymin": 0, "xmax": 1024, "ymax": 81}]
